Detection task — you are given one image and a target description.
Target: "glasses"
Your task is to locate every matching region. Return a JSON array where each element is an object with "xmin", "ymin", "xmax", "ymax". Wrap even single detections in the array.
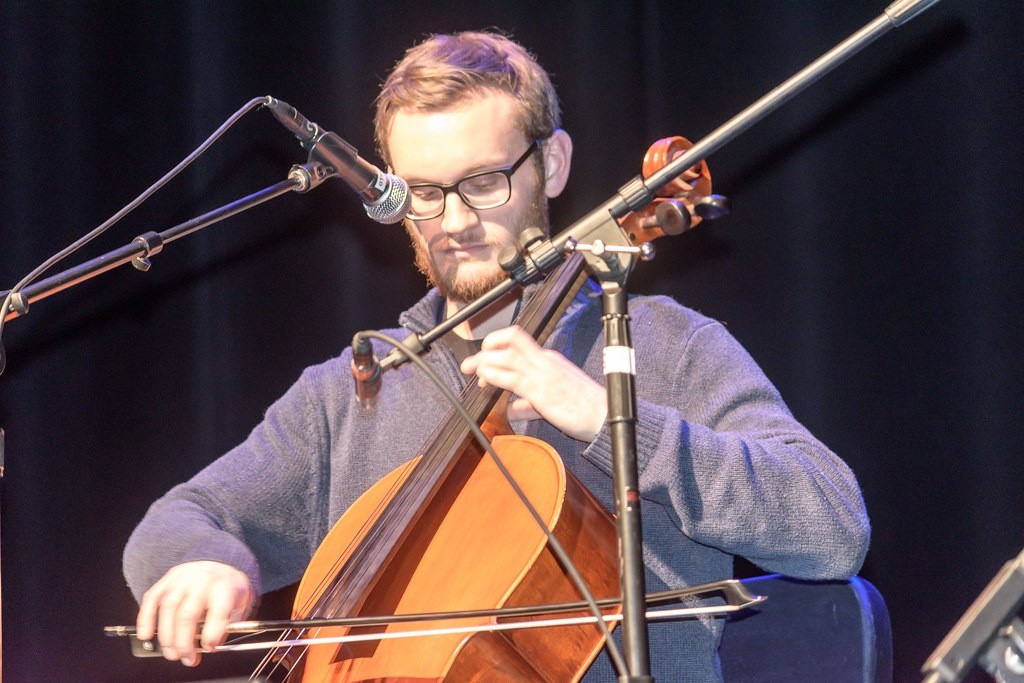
[{"xmin": 384, "ymin": 132, "xmax": 550, "ymax": 221}]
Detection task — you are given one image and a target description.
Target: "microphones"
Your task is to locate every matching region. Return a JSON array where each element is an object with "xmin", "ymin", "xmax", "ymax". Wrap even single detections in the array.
[
  {"xmin": 350, "ymin": 333, "xmax": 382, "ymax": 411},
  {"xmin": 262, "ymin": 94, "xmax": 412, "ymax": 225}
]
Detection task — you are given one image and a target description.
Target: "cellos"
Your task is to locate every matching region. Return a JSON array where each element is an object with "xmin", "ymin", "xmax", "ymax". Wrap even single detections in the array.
[{"xmin": 242, "ymin": 135, "xmax": 743, "ymax": 683}]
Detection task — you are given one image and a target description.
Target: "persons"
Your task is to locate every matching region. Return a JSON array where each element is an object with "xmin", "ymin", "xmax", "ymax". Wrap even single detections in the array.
[{"xmin": 123, "ymin": 32, "xmax": 872, "ymax": 682}]
